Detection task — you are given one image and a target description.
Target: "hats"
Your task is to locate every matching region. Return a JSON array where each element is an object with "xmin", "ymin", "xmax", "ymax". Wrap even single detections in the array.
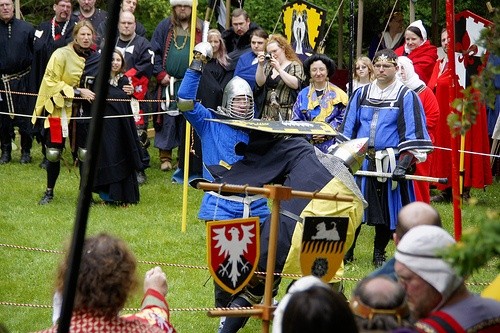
[{"xmin": 170, "ymin": 0, "xmax": 198, "ymax": 9}]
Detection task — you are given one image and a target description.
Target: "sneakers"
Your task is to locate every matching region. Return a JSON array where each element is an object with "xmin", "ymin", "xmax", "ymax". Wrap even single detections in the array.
[{"xmin": 39, "ymin": 190, "xmax": 53, "ymax": 204}]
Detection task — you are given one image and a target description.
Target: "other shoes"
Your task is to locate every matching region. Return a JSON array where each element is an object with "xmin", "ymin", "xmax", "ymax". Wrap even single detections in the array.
[
  {"xmin": 39, "ymin": 155, "xmax": 48, "ymax": 168},
  {"xmin": 136, "ymin": 170, "xmax": 145, "ymax": 184}
]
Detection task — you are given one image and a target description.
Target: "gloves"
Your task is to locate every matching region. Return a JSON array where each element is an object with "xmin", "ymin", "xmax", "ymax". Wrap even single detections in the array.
[{"xmin": 392, "ymin": 152, "xmax": 414, "ymax": 181}]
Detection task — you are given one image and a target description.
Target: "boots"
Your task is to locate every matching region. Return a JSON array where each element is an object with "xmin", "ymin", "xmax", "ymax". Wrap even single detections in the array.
[
  {"xmin": 20, "ymin": 147, "xmax": 31, "ymax": 162},
  {"xmin": 342, "ymin": 225, "xmax": 362, "ymax": 265},
  {"xmin": 373, "ymin": 231, "xmax": 391, "ymax": 267},
  {"xmin": 0, "ymin": 145, "xmax": 12, "ymax": 165},
  {"xmin": 160, "ymin": 148, "xmax": 172, "ymax": 170},
  {"xmin": 173, "ymin": 146, "xmax": 181, "ymax": 168}
]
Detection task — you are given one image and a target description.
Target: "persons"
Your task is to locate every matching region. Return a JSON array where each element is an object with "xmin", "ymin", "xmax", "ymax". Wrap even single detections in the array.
[
  {"xmin": 98, "ymin": 0, "xmax": 155, "ymax": 184},
  {"xmin": 34, "ymin": 0, "xmax": 108, "ymax": 167},
  {"xmin": 381, "ymin": 8, "xmax": 500, "ymax": 204},
  {"xmin": 77, "ymin": 50, "xmax": 145, "ymax": 206},
  {"xmin": 33, "ymin": 233, "xmax": 177, "ymax": 333},
  {"xmin": 234, "ymin": 30, "xmax": 306, "ymax": 121},
  {"xmin": 272, "ymin": 276, "xmax": 417, "ymax": 333},
  {"xmin": 152, "ymin": 0, "xmax": 204, "ymax": 169},
  {"xmin": 346, "ymin": 57, "xmax": 376, "ymax": 93},
  {"xmin": 0, "ymin": 0, "xmax": 35, "ymax": 163},
  {"xmin": 178, "ymin": 10, "xmax": 260, "ymax": 173},
  {"xmin": 190, "ymin": 138, "xmax": 369, "ymax": 333},
  {"xmin": 291, "ymin": 54, "xmax": 349, "ymax": 153},
  {"xmin": 341, "ymin": 49, "xmax": 434, "ymax": 267},
  {"xmin": 177, "ymin": 52, "xmax": 262, "ymax": 326},
  {"xmin": 31, "ymin": 22, "xmax": 134, "ymax": 205},
  {"xmin": 394, "ymin": 225, "xmax": 500, "ymax": 333},
  {"xmin": 374, "ymin": 201, "xmax": 443, "ymax": 282}
]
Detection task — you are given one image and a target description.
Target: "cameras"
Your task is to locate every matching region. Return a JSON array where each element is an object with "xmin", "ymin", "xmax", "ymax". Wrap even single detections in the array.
[{"xmin": 263, "ymin": 53, "xmax": 272, "ymax": 62}]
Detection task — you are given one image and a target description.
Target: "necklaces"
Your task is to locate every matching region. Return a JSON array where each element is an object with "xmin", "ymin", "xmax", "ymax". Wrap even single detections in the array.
[{"xmin": 173, "ymin": 25, "xmax": 189, "ymax": 49}]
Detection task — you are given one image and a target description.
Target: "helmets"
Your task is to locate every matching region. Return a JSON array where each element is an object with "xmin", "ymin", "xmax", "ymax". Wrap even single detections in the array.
[
  {"xmin": 222, "ymin": 76, "xmax": 254, "ymax": 121},
  {"xmin": 328, "ymin": 137, "xmax": 370, "ymax": 176}
]
